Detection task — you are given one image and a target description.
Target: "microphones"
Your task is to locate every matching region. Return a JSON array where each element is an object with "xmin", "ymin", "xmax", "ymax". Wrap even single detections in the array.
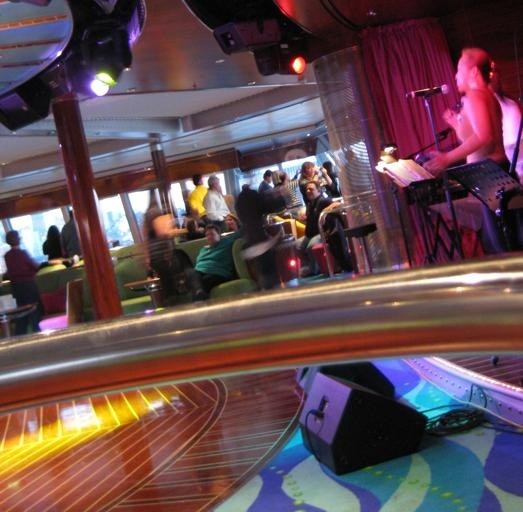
[{"xmin": 405, "ymin": 84, "xmax": 449, "ymax": 99}]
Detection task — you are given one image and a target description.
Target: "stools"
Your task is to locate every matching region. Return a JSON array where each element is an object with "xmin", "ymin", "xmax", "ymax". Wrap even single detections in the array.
[{"xmin": 339, "ymin": 220, "xmax": 377, "ymax": 278}]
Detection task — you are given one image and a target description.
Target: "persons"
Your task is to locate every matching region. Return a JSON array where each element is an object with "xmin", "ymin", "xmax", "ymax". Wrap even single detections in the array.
[
  {"xmin": 423, "ymin": 48, "xmax": 520, "ymax": 256},
  {"xmin": 43, "ymin": 225, "xmax": 62, "ymax": 260},
  {"xmin": 5, "ymin": 231, "xmax": 42, "ymax": 334},
  {"xmin": 144, "ymin": 151, "xmax": 376, "ymax": 300},
  {"xmin": 487, "ymin": 73, "xmax": 523, "ymax": 249},
  {"xmin": 59, "ymin": 210, "xmax": 113, "ymax": 261}
]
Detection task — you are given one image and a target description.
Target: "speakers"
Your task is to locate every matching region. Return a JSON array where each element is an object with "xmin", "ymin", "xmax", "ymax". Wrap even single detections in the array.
[
  {"xmin": 298, "ymin": 372, "xmax": 428, "ymax": 475},
  {"xmin": 293, "ymin": 361, "xmax": 395, "ymax": 400}
]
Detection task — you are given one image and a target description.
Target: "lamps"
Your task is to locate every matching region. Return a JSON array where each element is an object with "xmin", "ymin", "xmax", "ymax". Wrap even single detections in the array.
[
  {"xmin": 211, "ymin": 12, "xmax": 311, "ymax": 78},
  {"xmin": 0, "ymin": 0, "xmax": 151, "ymax": 140}
]
